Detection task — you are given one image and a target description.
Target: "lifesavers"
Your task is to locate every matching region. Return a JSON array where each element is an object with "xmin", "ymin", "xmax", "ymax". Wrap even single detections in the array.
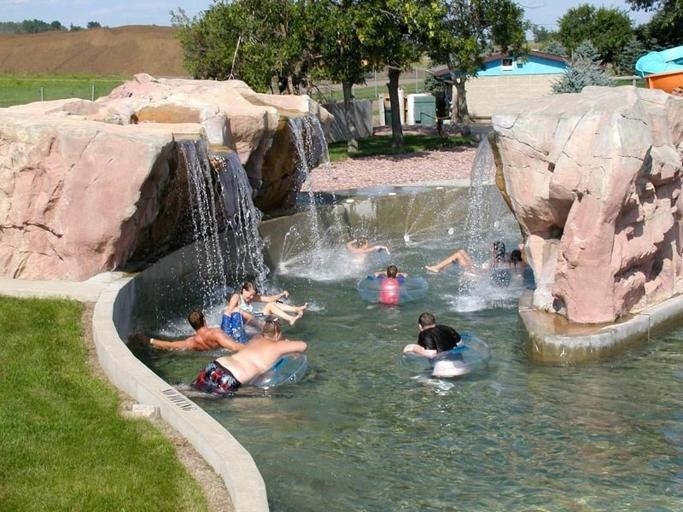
[
  {"xmin": 253, "ymin": 353, "xmax": 308, "ymax": 393},
  {"xmin": 400, "ymin": 332, "xmax": 490, "ymax": 376},
  {"xmin": 359, "ymin": 274, "xmax": 428, "ymax": 304}
]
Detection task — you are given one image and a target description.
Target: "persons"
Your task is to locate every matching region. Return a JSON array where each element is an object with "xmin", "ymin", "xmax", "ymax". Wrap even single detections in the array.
[
  {"xmin": 366, "ymin": 265, "xmax": 408, "ymax": 306},
  {"xmin": 402, "ymin": 312, "xmax": 465, "ymax": 382},
  {"xmin": 224, "ymin": 278, "xmax": 309, "ymax": 326},
  {"xmin": 148, "ymin": 309, "xmax": 268, "ymax": 355},
  {"xmin": 345, "ymin": 234, "xmax": 391, "ymax": 261},
  {"xmin": 425, "ymin": 241, "xmax": 526, "ymax": 288},
  {"xmin": 171, "ymin": 320, "xmax": 308, "ymax": 402}
]
